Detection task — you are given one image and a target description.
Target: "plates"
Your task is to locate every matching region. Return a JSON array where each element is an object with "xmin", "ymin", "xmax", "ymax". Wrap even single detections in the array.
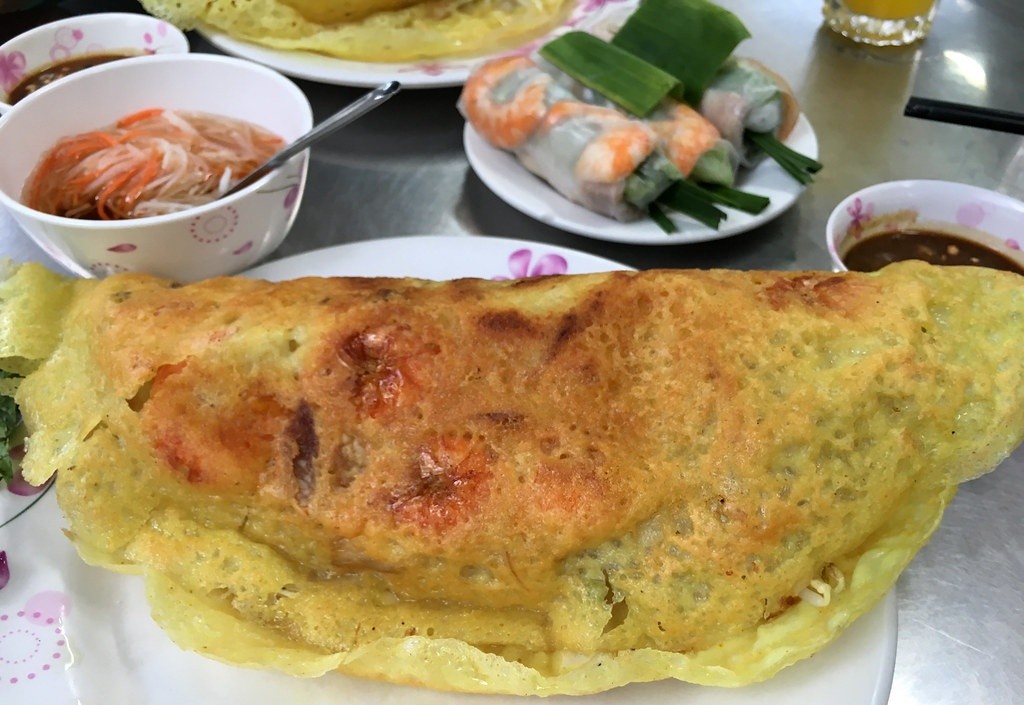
[
  {"xmin": 191, "ymin": 0, "xmax": 645, "ymax": 89},
  {"xmin": 0, "ymin": 237, "xmax": 900, "ymax": 705},
  {"xmin": 463, "ymin": 107, "xmax": 819, "ymax": 245}
]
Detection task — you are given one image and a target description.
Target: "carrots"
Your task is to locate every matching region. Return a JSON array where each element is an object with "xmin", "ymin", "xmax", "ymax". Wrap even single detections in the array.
[{"xmin": 23, "ymin": 108, "xmax": 283, "ymax": 218}]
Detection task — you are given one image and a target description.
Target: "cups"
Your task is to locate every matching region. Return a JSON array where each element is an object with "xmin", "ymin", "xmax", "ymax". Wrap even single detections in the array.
[{"xmin": 822, "ymin": 0, "xmax": 940, "ymax": 46}]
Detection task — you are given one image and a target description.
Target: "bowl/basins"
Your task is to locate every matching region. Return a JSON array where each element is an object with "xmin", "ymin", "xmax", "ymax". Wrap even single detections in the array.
[
  {"xmin": 0, "ymin": 53, "xmax": 312, "ymax": 285},
  {"xmin": 0, "ymin": 12, "xmax": 189, "ymax": 118},
  {"xmin": 826, "ymin": 179, "xmax": 1024, "ymax": 274}
]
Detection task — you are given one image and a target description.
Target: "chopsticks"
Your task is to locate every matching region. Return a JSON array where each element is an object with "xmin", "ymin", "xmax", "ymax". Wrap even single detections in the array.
[{"xmin": 904, "ymin": 95, "xmax": 1024, "ymax": 135}]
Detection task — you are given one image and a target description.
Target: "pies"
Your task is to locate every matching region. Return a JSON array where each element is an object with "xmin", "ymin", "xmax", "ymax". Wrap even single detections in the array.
[
  {"xmin": 135, "ymin": 0, "xmax": 573, "ymax": 66},
  {"xmin": 2, "ymin": 255, "xmax": 1024, "ymax": 694}
]
